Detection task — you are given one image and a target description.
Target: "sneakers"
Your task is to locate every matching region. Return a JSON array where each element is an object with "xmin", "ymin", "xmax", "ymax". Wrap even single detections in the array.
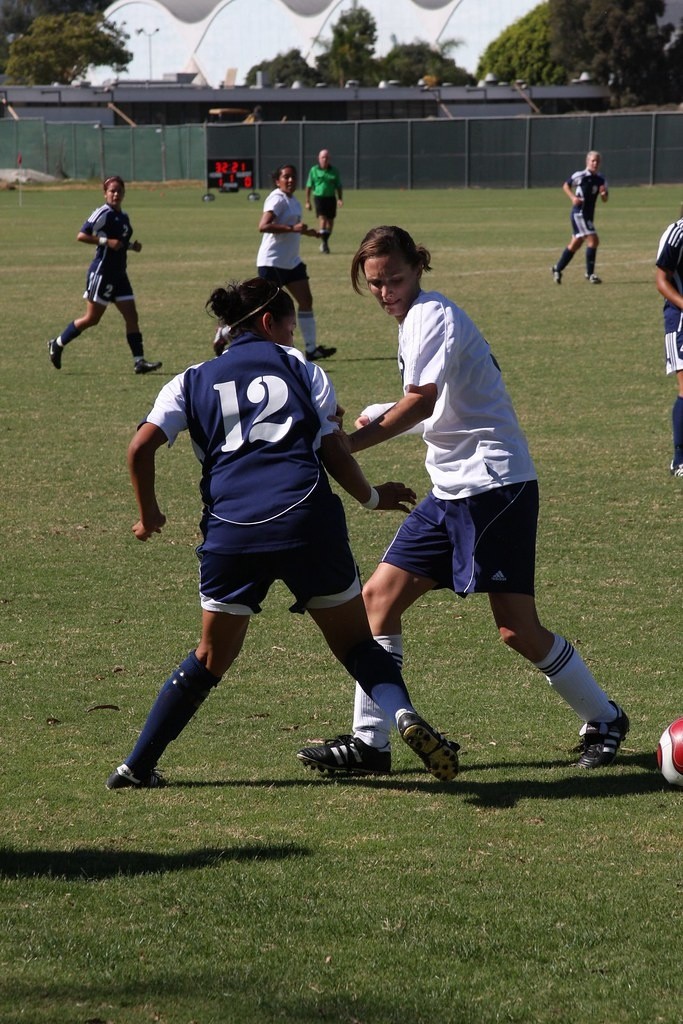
[
  {"xmin": 106, "ymin": 764, "xmax": 167, "ymax": 790},
  {"xmin": 568, "ymin": 700, "xmax": 630, "ymax": 768},
  {"xmin": 670, "ymin": 460, "xmax": 683, "ymax": 476},
  {"xmin": 212, "ymin": 325, "xmax": 228, "ymax": 357},
  {"xmin": 296, "ymin": 734, "xmax": 392, "ymax": 775},
  {"xmin": 134, "ymin": 359, "xmax": 161, "ymax": 374},
  {"xmin": 395, "ymin": 708, "xmax": 460, "ymax": 782},
  {"xmin": 47, "ymin": 338, "xmax": 64, "ymax": 369},
  {"xmin": 305, "ymin": 345, "xmax": 337, "ymax": 362}
]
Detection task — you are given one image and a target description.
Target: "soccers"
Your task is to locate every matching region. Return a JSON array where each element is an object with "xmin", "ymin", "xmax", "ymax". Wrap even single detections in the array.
[{"xmin": 656, "ymin": 717, "xmax": 683, "ymax": 786}]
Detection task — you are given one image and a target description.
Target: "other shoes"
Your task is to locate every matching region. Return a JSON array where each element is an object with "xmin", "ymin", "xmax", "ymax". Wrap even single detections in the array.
[
  {"xmin": 584, "ymin": 272, "xmax": 601, "ymax": 284},
  {"xmin": 552, "ymin": 266, "xmax": 561, "ymax": 284},
  {"xmin": 320, "ymin": 243, "xmax": 329, "ymax": 254}
]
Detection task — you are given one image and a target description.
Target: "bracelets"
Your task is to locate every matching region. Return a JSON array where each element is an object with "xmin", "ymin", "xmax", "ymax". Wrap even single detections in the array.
[
  {"xmin": 362, "ymin": 489, "xmax": 380, "ymax": 509},
  {"xmin": 98, "ymin": 237, "xmax": 108, "ymax": 246}
]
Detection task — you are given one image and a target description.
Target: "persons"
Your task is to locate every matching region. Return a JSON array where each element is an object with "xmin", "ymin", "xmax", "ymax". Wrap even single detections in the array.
[
  {"xmin": 294, "ymin": 224, "xmax": 629, "ymax": 776},
  {"xmin": 655, "ymin": 214, "xmax": 683, "ymax": 479},
  {"xmin": 106, "ymin": 277, "xmax": 461, "ymax": 789},
  {"xmin": 305, "ymin": 149, "xmax": 344, "ymax": 253},
  {"xmin": 48, "ymin": 177, "xmax": 163, "ymax": 375},
  {"xmin": 551, "ymin": 151, "xmax": 609, "ymax": 285},
  {"xmin": 213, "ymin": 163, "xmax": 337, "ymax": 361}
]
